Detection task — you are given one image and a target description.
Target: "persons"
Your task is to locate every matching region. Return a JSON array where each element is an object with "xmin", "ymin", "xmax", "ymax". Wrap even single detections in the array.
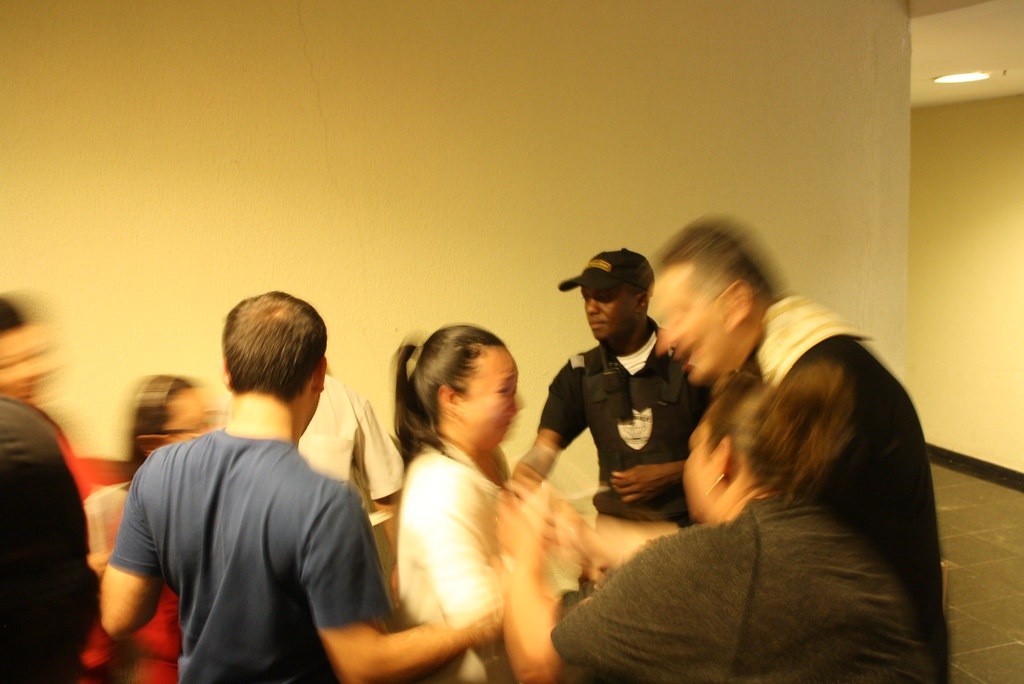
[
  {"xmin": 101, "ymin": 291, "xmax": 510, "ymax": 684},
  {"xmin": 391, "ymin": 220, "xmax": 942, "ymax": 684},
  {"xmin": 0, "ymin": 291, "xmax": 214, "ymax": 684},
  {"xmin": 297, "ymin": 373, "xmax": 403, "ymax": 558}
]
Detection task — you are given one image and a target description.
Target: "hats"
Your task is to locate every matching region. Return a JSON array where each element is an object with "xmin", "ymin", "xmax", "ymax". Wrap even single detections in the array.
[{"xmin": 559, "ymin": 248, "xmax": 655, "ymax": 291}]
